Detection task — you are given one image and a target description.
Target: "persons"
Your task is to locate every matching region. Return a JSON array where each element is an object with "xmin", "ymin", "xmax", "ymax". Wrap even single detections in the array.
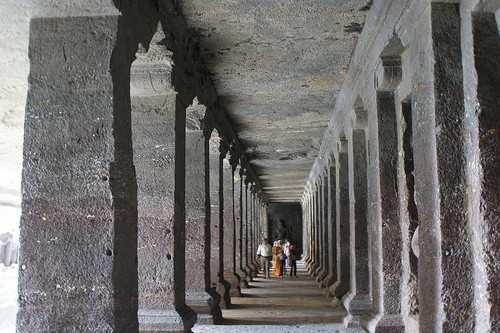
[
  {"xmin": 257, "ymin": 238, "xmax": 272, "ymax": 278},
  {"xmin": 289, "ymin": 245, "xmax": 299, "ymax": 277},
  {"xmin": 282, "ymin": 239, "xmax": 291, "ymax": 267},
  {"xmin": 277, "ymin": 249, "xmax": 288, "ymax": 278},
  {"xmin": 272, "ymin": 242, "xmax": 282, "ymax": 275}
]
